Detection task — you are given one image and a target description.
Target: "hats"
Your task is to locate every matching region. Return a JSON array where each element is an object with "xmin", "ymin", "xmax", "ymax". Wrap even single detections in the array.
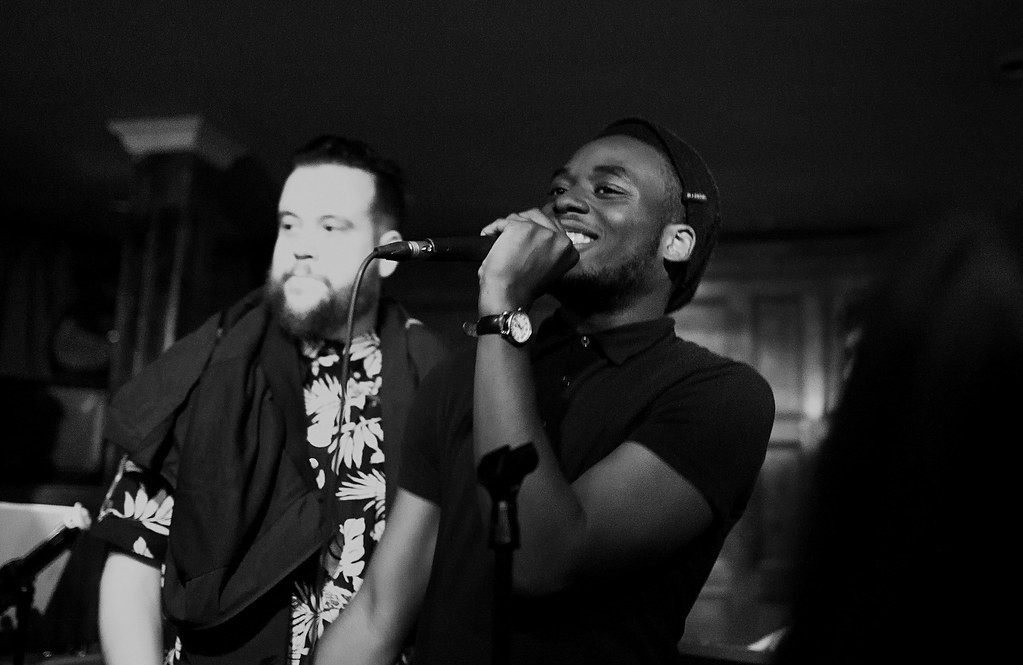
[{"xmin": 604, "ymin": 116, "xmax": 722, "ymax": 315}]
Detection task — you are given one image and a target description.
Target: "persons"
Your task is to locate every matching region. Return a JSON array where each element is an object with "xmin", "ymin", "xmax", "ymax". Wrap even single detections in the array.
[
  {"xmin": 309, "ymin": 118, "xmax": 777, "ymax": 662},
  {"xmin": 92, "ymin": 133, "xmax": 447, "ymax": 665},
  {"xmin": 747, "ymin": 56, "xmax": 1022, "ymax": 665}
]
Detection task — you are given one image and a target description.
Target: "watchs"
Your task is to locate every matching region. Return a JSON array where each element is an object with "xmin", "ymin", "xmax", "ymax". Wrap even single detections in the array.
[{"xmin": 462, "ymin": 306, "xmax": 534, "ymax": 349}]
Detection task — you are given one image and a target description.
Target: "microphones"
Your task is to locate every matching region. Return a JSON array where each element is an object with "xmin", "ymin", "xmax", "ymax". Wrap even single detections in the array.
[{"xmin": 374, "ymin": 230, "xmax": 502, "ymax": 264}]
